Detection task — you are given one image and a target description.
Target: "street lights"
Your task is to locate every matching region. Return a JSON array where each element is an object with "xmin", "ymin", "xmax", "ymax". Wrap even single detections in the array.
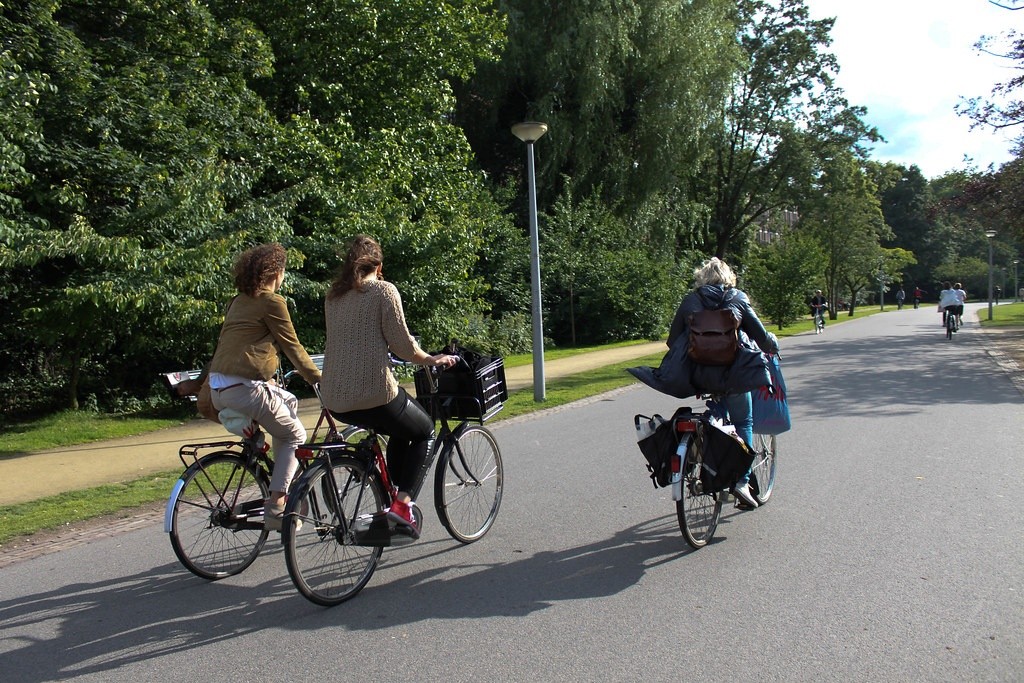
[
  {"xmin": 985, "ymin": 230, "xmax": 997, "ymax": 320},
  {"xmin": 1013, "ymin": 260, "xmax": 1019, "ymax": 302},
  {"xmin": 1002, "ymin": 269, "xmax": 1006, "ymax": 299},
  {"xmin": 511, "ymin": 122, "xmax": 550, "ymax": 400}
]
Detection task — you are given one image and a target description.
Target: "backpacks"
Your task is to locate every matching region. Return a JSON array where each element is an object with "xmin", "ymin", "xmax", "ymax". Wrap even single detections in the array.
[{"xmin": 687, "ymin": 308, "xmax": 739, "ymax": 367}]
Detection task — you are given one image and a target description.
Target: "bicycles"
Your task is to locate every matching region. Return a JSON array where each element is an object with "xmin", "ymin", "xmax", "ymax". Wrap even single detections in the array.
[
  {"xmin": 812, "ymin": 305, "xmax": 826, "ymax": 334},
  {"xmin": 671, "ymin": 349, "xmax": 778, "ymax": 550},
  {"xmin": 898, "ymin": 299, "xmax": 903, "ymax": 309},
  {"xmin": 163, "ymin": 369, "xmax": 388, "ymax": 584},
  {"xmin": 945, "ymin": 310, "xmax": 953, "ymax": 339},
  {"xmin": 279, "ymin": 351, "xmax": 506, "ymax": 607},
  {"xmin": 914, "ymin": 298, "xmax": 918, "ymax": 308}
]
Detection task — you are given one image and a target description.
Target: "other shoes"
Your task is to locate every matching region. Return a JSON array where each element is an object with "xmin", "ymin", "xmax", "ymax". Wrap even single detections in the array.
[
  {"xmin": 955, "ymin": 323, "xmax": 959, "ymax": 329},
  {"xmin": 959, "ymin": 318, "xmax": 963, "ymax": 325}
]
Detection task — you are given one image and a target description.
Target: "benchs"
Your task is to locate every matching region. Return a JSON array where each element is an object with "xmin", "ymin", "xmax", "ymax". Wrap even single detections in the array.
[
  {"xmin": 309, "ymin": 354, "xmax": 325, "ymax": 374},
  {"xmin": 155, "ymin": 370, "xmax": 207, "ymax": 420}
]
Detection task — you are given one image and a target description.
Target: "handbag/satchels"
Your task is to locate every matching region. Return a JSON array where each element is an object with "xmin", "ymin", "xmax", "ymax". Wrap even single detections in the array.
[
  {"xmin": 938, "ymin": 301, "xmax": 943, "ymax": 312},
  {"xmin": 751, "ymin": 354, "xmax": 791, "ymax": 435},
  {"xmin": 635, "ymin": 406, "xmax": 697, "ymax": 489},
  {"xmin": 196, "ymin": 374, "xmax": 222, "ymax": 424},
  {"xmin": 700, "ymin": 411, "xmax": 758, "ymax": 495}
]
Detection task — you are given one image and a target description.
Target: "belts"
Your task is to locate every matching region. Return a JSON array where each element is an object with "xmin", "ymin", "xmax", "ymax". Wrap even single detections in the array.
[{"xmin": 212, "ymin": 383, "xmax": 244, "ymax": 393}]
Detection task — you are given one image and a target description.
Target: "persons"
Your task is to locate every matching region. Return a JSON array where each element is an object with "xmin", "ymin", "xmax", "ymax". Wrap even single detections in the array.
[
  {"xmin": 894, "ymin": 287, "xmax": 906, "ymax": 308},
  {"xmin": 994, "ymin": 286, "xmax": 1000, "ymax": 305},
  {"xmin": 912, "ymin": 286, "xmax": 928, "ymax": 306},
  {"xmin": 808, "ymin": 289, "xmax": 829, "ymax": 328},
  {"xmin": 1018, "ymin": 285, "xmax": 1024, "ymax": 302},
  {"xmin": 626, "ymin": 255, "xmax": 780, "ymax": 509},
  {"xmin": 208, "ymin": 242, "xmax": 324, "ymax": 533},
  {"xmin": 319, "ymin": 234, "xmax": 458, "ymax": 541},
  {"xmin": 938, "ymin": 281, "xmax": 967, "ymax": 333}
]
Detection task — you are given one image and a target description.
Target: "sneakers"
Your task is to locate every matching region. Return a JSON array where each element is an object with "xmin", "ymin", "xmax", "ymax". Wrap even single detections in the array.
[
  {"xmin": 733, "ymin": 481, "xmax": 759, "ymax": 508},
  {"xmin": 265, "ymin": 502, "xmax": 303, "ymax": 531},
  {"xmin": 387, "ymin": 498, "xmax": 420, "ymax": 540}
]
